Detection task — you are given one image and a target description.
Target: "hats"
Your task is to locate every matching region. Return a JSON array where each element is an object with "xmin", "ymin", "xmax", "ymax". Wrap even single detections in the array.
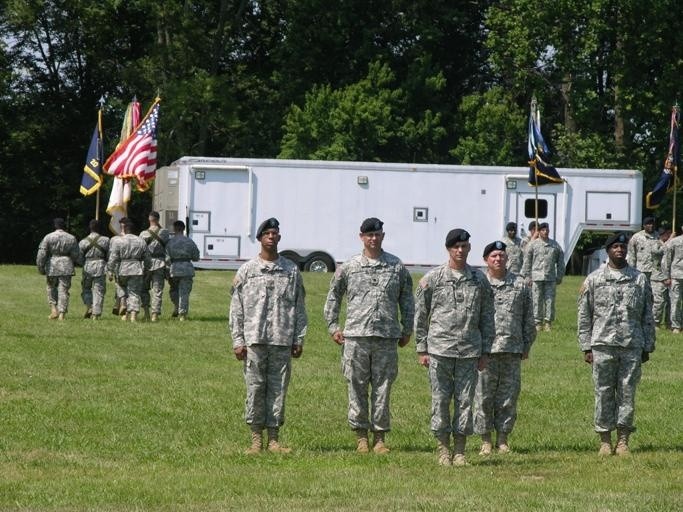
[
  {"xmin": 54, "ymin": 218, "xmax": 64, "ymax": 223},
  {"xmin": 484, "ymin": 241, "xmax": 506, "ymax": 256},
  {"xmin": 257, "ymin": 218, "xmax": 279, "ymax": 237},
  {"xmin": 361, "ymin": 218, "xmax": 383, "ymax": 232},
  {"xmin": 506, "ymin": 221, "xmax": 549, "ymax": 230},
  {"xmin": 150, "ymin": 212, "xmax": 158, "ymax": 216},
  {"xmin": 119, "ymin": 217, "xmax": 136, "ymax": 222},
  {"xmin": 175, "ymin": 221, "xmax": 183, "ymax": 226},
  {"xmin": 445, "ymin": 229, "xmax": 470, "ymax": 246},
  {"xmin": 90, "ymin": 220, "xmax": 101, "ymax": 224},
  {"xmin": 606, "ymin": 232, "xmax": 627, "ymax": 249},
  {"xmin": 643, "ymin": 217, "xmax": 652, "ymax": 224}
]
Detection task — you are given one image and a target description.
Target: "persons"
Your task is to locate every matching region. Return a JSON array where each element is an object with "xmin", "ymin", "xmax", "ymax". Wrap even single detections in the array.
[
  {"xmin": 229, "ymin": 218, "xmax": 307, "ymax": 453},
  {"xmin": 473, "ymin": 241, "xmax": 537, "ymax": 455},
  {"xmin": 323, "ymin": 217, "xmax": 415, "ymax": 453},
  {"xmin": 626, "ymin": 217, "xmax": 683, "ymax": 333},
  {"xmin": 500, "ymin": 221, "xmax": 566, "ymax": 333},
  {"xmin": 36, "ymin": 211, "xmax": 200, "ymax": 323},
  {"xmin": 576, "ymin": 233, "xmax": 656, "ymax": 455},
  {"xmin": 414, "ymin": 229, "xmax": 496, "ymax": 466}
]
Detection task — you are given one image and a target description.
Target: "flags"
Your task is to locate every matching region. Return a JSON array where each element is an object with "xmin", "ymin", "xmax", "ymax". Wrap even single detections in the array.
[
  {"xmin": 102, "ymin": 97, "xmax": 161, "ymax": 193},
  {"xmin": 80, "ymin": 109, "xmax": 105, "ymax": 197},
  {"xmin": 646, "ymin": 105, "xmax": 681, "ymax": 209},
  {"xmin": 527, "ymin": 99, "xmax": 564, "ymax": 187},
  {"xmin": 105, "ymin": 102, "xmax": 140, "ymax": 236}
]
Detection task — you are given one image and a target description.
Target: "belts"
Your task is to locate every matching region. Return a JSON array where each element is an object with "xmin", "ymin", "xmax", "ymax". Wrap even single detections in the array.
[
  {"xmin": 121, "ymin": 258, "xmax": 139, "ymax": 261},
  {"xmin": 86, "ymin": 257, "xmax": 104, "ymax": 260},
  {"xmin": 173, "ymin": 259, "xmax": 190, "ymax": 262},
  {"xmin": 50, "ymin": 253, "xmax": 70, "ymax": 257},
  {"xmin": 152, "ymin": 254, "xmax": 164, "ymax": 258}
]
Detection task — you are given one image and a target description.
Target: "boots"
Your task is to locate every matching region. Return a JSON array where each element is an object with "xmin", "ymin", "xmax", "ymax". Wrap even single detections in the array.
[
  {"xmin": 356, "ymin": 429, "xmax": 369, "ymax": 453},
  {"xmin": 479, "ymin": 437, "xmax": 492, "ymax": 456},
  {"xmin": 267, "ymin": 428, "xmax": 290, "ymax": 453},
  {"xmin": 85, "ymin": 304, "xmax": 97, "ymax": 320},
  {"xmin": 615, "ymin": 435, "xmax": 629, "ymax": 454},
  {"xmin": 496, "ymin": 433, "xmax": 512, "ymax": 453},
  {"xmin": 536, "ymin": 322, "xmax": 551, "ymax": 331},
  {"xmin": 113, "ymin": 303, "xmax": 136, "ymax": 321},
  {"xmin": 48, "ymin": 305, "xmax": 64, "ymax": 320},
  {"xmin": 598, "ymin": 433, "xmax": 611, "ymax": 456},
  {"xmin": 243, "ymin": 428, "xmax": 262, "ymax": 455},
  {"xmin": 372, "ymin": 433, "xmax": 389, "ymax": 454},
  {"xmin": 172, "ymin": 305, "xmax": 185, "ymax": 321},
  {"xmin": 452, "ymin": 434, "xmax": 467, "ymax": 465},
  {"xmin": 438, "ymin": 433, "xmax": 452, "ymax": 465},
  {"xmin": 144, "ymin": 306, "xmax": 159, "ymax": 321}
]
